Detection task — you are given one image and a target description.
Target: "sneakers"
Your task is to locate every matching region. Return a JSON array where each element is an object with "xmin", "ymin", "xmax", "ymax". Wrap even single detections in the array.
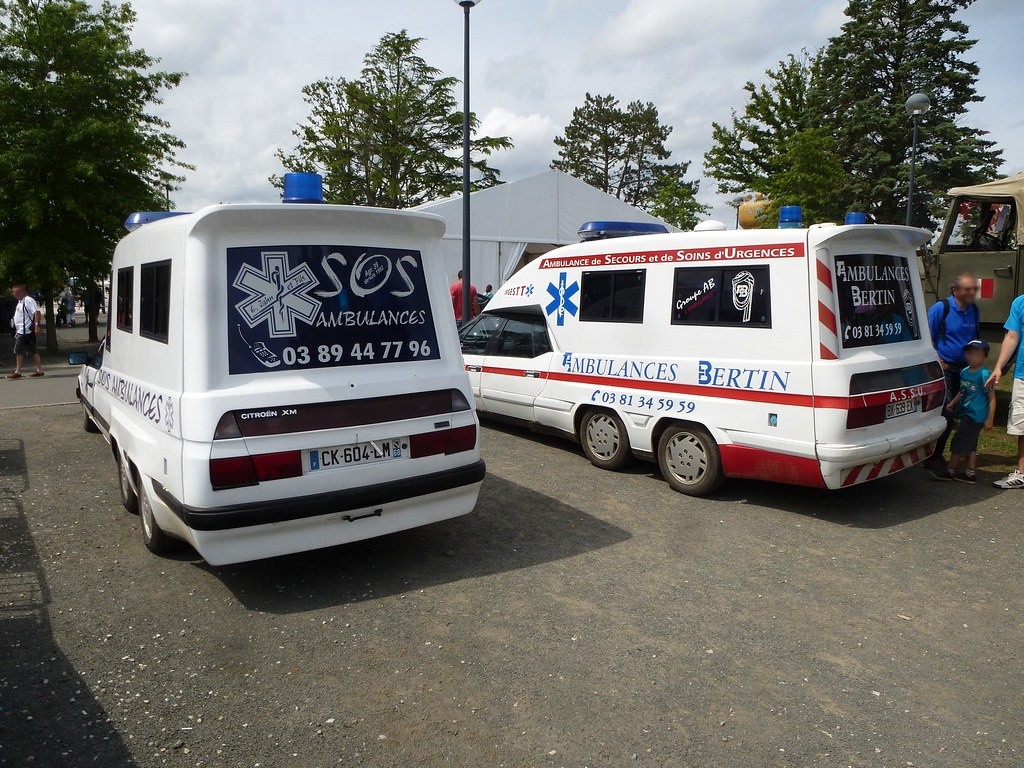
[{"xmin": 992, "ymin": 470, "xmax": 1024, "ymax": 489}]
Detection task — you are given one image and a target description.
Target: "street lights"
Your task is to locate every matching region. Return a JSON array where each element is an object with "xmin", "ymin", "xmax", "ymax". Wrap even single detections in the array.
[
  {"xmin": 453, "ymin": 0, "xmax": 484, "ymax": 324},
  {"xmin": 899, "ymin": 92, "xmax": 935, "ymax": 226}
]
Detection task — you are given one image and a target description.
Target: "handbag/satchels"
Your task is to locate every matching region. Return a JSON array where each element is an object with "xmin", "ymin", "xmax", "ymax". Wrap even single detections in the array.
[{"xmin": 29, "ymin": 322, "xmax": 35, "ymax": 332}]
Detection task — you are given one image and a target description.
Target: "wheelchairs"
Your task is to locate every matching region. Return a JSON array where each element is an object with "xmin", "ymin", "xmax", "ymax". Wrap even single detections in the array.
[{"xmin": 54, "ymin": 302, "xmax": 77, "ymax": 328}]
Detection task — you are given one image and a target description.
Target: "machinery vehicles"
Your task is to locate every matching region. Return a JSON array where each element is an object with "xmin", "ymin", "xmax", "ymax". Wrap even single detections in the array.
[{"xmin": 914, "ymin": 172, "xmax": 1024, "ymax": 408}]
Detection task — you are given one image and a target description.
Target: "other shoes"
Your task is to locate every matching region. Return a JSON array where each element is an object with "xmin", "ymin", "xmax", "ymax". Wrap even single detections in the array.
[
  {"xmin": 952, "ymin": 472, "xmax": 976, "ymax": 484},
  {"xmin": 928, "ymin": 470, "xmax": 953, "ymax": 481},
  {"xmin": 5, "ymin": 373, "xmax": 21, "ymax": 379},
  {"xmin": 27, "ymin": 371, "xmax": 45, "ymax": 379}
]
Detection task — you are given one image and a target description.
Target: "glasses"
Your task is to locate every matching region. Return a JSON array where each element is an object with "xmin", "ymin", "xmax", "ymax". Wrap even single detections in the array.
[{"xmin": 955, "ymin": 287, "xmax": 979, "ymax": 292}]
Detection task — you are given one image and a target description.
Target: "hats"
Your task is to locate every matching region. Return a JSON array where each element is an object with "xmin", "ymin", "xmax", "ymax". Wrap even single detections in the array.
[{"xmin": 961, "ymin": 339, "xmax": 990, "ymax": 356}]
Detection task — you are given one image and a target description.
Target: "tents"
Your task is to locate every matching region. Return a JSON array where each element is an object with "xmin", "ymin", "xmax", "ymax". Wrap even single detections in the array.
[{"xmin": 409, "ymin": 170, "xmax": 683, "ymax": 293}]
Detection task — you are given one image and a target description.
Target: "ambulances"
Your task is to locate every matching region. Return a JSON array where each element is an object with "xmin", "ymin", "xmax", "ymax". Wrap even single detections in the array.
[
  {"xmin": 67, "ymin": 171, "xmax": 486, "ymax": 571},
  {"xmin": 457, "ymin": 209, "xmax": 948, "ymax": 501}
]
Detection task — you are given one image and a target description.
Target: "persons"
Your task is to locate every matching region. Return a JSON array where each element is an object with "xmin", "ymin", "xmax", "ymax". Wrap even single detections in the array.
[
  {"xmin": 926, "ymin": 338, "xmax": 995, "ymax": 484},
  {"xmin": 479, "ymin": 285, "xmax": 493, "ymax": 304},
  {"xmin": 5, "ymin": 286, "xmax": 44, "ymax": 379},
  {"xmin": 80, "ymin": 286, "xmax": 100, "ymax": 324},
  {"xmin": 451, "ymin": 269, "xmax": 480, "ymax": 326},
  {"xmin": 982, "ymin": 293, "xmax": 1024, "ymax": 488},
  {"xmin": 61, "ymin": 291, "xmax": 76, "ymax": 324},
  {"xmin": 929, "ymin": 274, "xmax": 981, "ymax": 465}
]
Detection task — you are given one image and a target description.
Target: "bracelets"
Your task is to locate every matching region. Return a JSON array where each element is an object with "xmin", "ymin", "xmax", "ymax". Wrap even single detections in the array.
[{"xmin": 35, "ymin": 323, "xmax": 39, "ymax": 326}]
